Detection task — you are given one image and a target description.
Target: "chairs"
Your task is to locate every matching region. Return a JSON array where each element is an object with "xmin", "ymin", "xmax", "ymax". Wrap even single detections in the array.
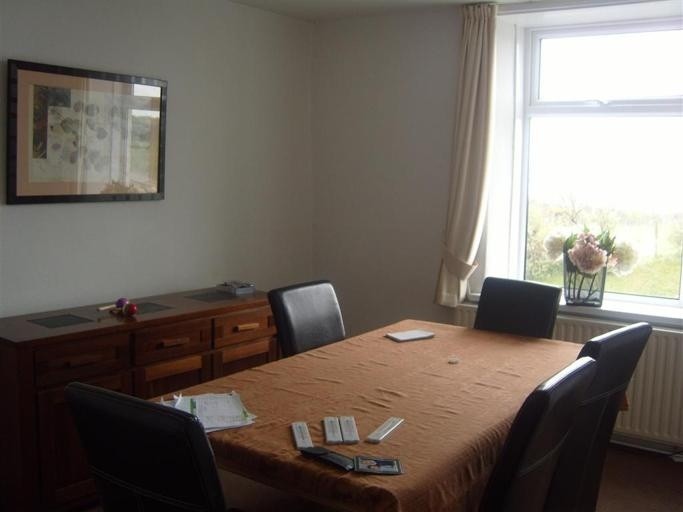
[
  {"xmin": 474, "ymin": 278, "xmax": 562, "ymax": 339},
  {"xmin": 479, "ymin": 357, "xmax": 594, "ymax": 512},
  {"xmin": 59, "ymin": 381, "xmax": 227, "ymax": 512},
  {"xmin": 546, "ymin": 321, "xmax": 651, "ymax": 512},
  {"xmin": 267, "ymin": 279, "xmax": 346, "ymax": 357}
]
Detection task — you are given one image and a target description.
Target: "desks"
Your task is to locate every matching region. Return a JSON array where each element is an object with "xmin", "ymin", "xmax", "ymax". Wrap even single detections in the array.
[{"xmin": 146, "ymin": 316, "xmax": 629, "ymax": 512}]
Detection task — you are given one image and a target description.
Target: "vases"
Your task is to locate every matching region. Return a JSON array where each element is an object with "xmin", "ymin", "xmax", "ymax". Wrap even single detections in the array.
[{"xmin": 564, "ymin": 253, "xmax": 606, "ymax": 307}]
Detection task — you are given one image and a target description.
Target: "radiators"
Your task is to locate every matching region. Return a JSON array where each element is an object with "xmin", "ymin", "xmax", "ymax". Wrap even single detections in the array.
[{"xmin": 454, "ymin": 304, "xmax": 683, "ymax": 456}]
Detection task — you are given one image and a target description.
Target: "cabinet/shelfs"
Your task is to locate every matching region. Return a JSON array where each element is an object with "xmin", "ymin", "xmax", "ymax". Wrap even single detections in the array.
[{"xmin": 0, "ymin": 286, "xmax": 282, "ymax": 512}]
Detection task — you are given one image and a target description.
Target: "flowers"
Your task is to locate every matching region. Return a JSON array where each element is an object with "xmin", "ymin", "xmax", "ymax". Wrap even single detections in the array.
[{"xmin": 544, "ymin": 226, "xmax": 638, "ymax": 277}]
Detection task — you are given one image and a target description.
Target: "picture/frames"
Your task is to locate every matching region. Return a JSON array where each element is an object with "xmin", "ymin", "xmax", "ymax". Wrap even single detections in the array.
[
  {"xmin": 7, "ymin": 60, "xmax": 167, "ymax": 204},
  {"xmin": 354, "ymin": 456, "xmax": 401, "ymax": 475}
]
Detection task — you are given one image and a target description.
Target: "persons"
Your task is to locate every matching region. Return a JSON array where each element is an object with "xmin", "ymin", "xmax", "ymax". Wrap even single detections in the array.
[{"xmin": 360, "ymin": 459, "xmax": 396, "ymax": 472}]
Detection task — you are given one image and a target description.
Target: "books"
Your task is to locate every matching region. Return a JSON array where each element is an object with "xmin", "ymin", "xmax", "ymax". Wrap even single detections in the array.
[
  {"xmin": 215, "ymin": 284, "xmax": 254, "ymax": 296},
  {"xmin": 385, "ymin": 329, "xmax": 434, "ymax": 342}
]
